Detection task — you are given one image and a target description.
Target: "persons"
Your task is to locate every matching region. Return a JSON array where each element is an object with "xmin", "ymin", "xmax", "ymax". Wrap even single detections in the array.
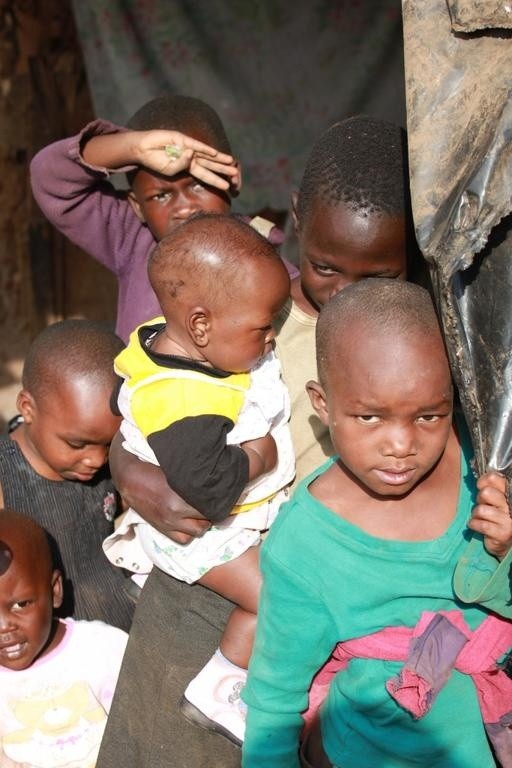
[
  {"xmin": 93, "ymin": 113, "xmax": 414, "ymax": 767},
  {"xmin": 1, "ymin": 316, "xmax": 143, "ymax": 635},
  {"xmin": 240, "ymin": 276, "xmax": 512, "ymax": 768},
  {"xmin": 106, "ymin": 210, "xmax": 296, "ymax": 748},
  {"xmin": 28, "ymin": 87, "xmax": 303, "ymax": 351},
  {"xmin": 0, "ymin": 506, "xmax": 130, "ymax": 768}
]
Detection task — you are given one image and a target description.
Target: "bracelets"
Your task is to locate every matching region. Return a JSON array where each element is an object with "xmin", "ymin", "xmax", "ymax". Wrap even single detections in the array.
[{"xmin": 243, "ymin": 442, "xmax": 269, "ymax": 471}]
